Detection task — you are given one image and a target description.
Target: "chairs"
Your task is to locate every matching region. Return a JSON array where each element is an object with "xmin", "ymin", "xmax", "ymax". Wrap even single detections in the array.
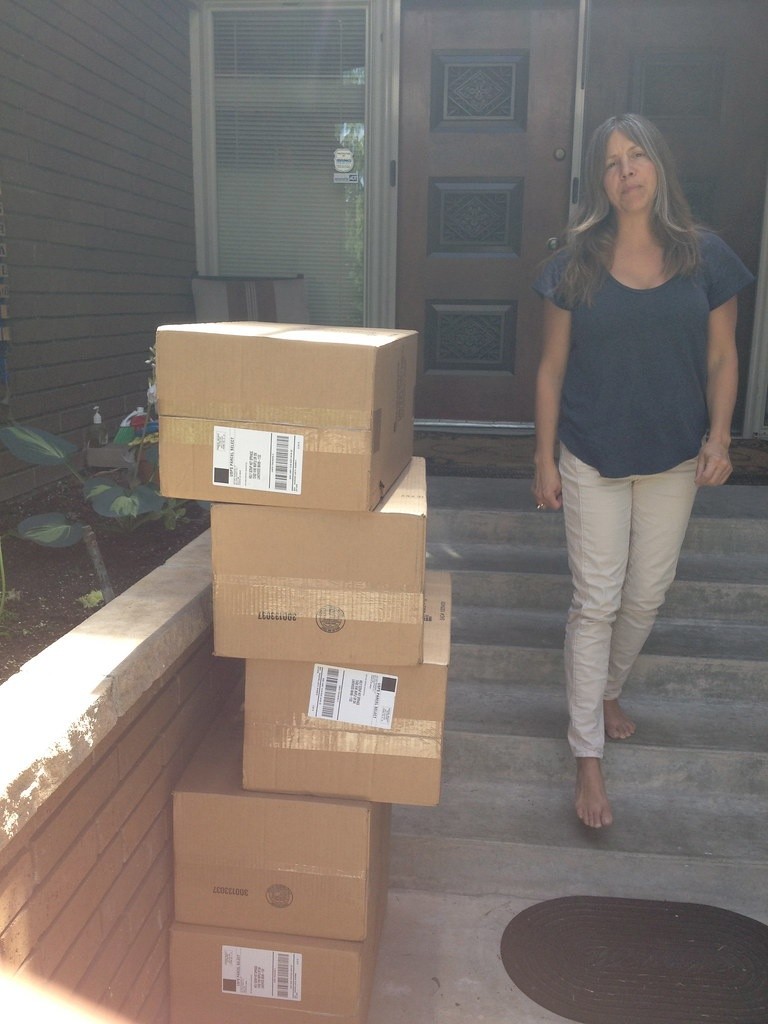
[{"xmin": 191, "ymin": 272, "xmax": 311, "ymax": 325}]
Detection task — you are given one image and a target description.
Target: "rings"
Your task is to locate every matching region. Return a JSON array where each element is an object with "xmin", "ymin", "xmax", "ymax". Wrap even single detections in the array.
[{"xmin": 537, "ymin": 503, "xmax": 544, "ymax": 509}]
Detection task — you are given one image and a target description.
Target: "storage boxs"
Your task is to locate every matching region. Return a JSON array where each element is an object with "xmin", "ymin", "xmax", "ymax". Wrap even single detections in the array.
[
  {"xmin": 155, "ymin": 319, "xmax": 419, "ymax": 512},
  {"xmin": 243, "ymin": 570, "xmax": 453, "ymax": 807},
  {"xmin": 170, "ymin": 675, "xmax": 392, "ymax": 941},
  {"xmin": 207, "ymin": 456, "xmax": 428, "ymax": 666},
  {"xmin": 170, "ymin": 883, "xmax": 389, "ymax": 1024}
]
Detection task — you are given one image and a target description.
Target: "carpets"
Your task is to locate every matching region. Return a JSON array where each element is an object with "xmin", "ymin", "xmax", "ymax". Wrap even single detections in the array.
[{"xmin": 501, "ymin": 896, "xmax": 768, "ymax": 1024}]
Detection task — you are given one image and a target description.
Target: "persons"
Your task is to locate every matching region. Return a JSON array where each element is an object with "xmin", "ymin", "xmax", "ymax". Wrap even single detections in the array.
[{"xmin": 530, "ymin": 112, "xmax": 756, "ymax": 829}]
